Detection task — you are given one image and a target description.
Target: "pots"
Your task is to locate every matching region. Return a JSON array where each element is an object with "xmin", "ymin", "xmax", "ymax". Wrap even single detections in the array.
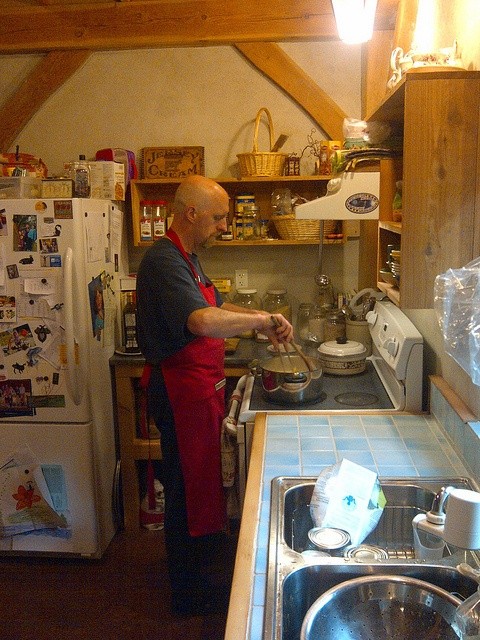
[
  {"xmin": 316, "ymin": 336, "xmax": 367, "ymax": 376},
  {"xmin": 248, "ymin": 355, "xmax": 325, "ymax": 404}
]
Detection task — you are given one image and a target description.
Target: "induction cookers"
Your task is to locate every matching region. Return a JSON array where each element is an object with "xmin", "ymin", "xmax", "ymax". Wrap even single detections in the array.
[{"xmin": 240, "ymin": 299, "xmax": 422, "ymax": 423}]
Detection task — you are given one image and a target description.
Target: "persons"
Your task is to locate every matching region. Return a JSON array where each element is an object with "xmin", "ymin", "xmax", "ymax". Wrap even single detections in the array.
[{"xmin": 134, "ymin": 175, "xmax": 292, "ymax": 619}]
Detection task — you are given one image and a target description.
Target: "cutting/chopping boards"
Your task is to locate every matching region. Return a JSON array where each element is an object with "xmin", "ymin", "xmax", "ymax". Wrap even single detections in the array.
[{"xmin": 224, "ymin": 336, "xmax": 240, "ymax": 355}]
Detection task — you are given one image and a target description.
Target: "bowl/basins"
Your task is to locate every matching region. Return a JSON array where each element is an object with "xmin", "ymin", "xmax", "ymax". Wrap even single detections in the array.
[
  {"xmin": 379, "ymin": 243, "xmax": 401, "ymax": 290},
  {"xmin": 300, "ymin": 573, "xmax": 465, "ymax": 640}
]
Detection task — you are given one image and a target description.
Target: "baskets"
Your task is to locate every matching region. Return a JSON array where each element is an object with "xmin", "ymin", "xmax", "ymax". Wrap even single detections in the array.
[
  {"xmin": 271, "ymin": 214, "xmax": 338, "ymax": 241},
  {"xmin": 235, "ymin": 107, "xmax": 289, "ymax": 178}
]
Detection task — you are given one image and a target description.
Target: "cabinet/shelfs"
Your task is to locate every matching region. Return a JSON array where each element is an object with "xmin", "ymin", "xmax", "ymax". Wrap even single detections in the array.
[
  {"xmin": 129, "ymin": 175, "xmax": 347, "ymax": 248},
  {"xmin": 364, "ymin": 71, "xmax": 480, "ymax": 309},
  {"xmin": 114, "ymin": 364, "xmax": 253, "ymax": 533}
]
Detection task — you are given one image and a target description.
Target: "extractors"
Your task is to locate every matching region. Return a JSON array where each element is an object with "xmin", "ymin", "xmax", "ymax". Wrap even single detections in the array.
[{"xmin": 292, "ymin": 171, "xmax": 382, "ymax": 221}]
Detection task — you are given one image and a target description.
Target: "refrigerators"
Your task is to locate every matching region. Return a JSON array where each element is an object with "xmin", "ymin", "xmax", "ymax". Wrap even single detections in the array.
[{"xmin": 1, "ymin": 197, "xmax": 128, "ymax": 562}]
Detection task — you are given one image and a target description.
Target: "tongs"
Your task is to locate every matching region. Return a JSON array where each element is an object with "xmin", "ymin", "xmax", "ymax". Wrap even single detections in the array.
[{"xmin": 270, "ymin": 315, "xmax": 317, "ymax": 372}]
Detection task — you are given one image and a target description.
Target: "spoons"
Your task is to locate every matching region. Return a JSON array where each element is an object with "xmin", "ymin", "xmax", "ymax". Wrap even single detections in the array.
[{"xmin": 315, "ymin": 219, "xmax": 331, "ymax": 288}]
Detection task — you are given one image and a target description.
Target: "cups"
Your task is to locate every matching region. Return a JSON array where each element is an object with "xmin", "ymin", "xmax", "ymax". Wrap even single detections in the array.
[{"xmin": 345, "ymin": 317, "xmax": 372, "ymax": 355}]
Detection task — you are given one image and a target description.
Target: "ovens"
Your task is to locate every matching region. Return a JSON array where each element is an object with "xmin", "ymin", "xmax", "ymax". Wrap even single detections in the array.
[{"xmin": 225, "ymin": 375, "xmax": 256, "ymax": 517}]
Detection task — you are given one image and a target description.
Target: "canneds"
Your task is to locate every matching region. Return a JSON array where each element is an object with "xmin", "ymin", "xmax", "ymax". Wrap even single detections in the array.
[
  {"xmin": 307, "ymin": 527, "xmax": 351, "ymax": 556},
  {"xmin": 344, "ymin": 543, "xmax": 388, "ymax": 560}
]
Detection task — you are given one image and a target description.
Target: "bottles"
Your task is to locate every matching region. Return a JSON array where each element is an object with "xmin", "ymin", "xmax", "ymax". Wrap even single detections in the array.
[
  {"xmin": 140, "ymin": 198, "xmax": 153, "ymax": 242},
  {"xmin": 122, "ymin": 291, "xmax": 138, "ymax": 354},
  {"xmin": 231, "ymin": 289, "xmax": 261, "ymax": 339},
  {"xmin": 297, "ymin": 303, "xmax": 312, "ymax": 330},
  {"xmin": 262, "ymin": 289, "xmax": 292, "ymax": 327},
  {"xmin": 72, "ymin": 154, "xmax": 92, "ymax": 198},
  {"xmin": 308, "ymin": 308, "xmax": 327, "ymax": 341},
  {"xmin": 325, "ymin": 312, "xmax": 345, "ymax": 341},
  {"xmin": 232, "ymin": 192, "xmax": 269, "ymax": 240},
  {"xmin": 391, "ymin": 179, "xmax": 403, "ymax": 222},
  {"xmin": 152, "ymin": 201, "xmax": 165, "ymax": 241}
]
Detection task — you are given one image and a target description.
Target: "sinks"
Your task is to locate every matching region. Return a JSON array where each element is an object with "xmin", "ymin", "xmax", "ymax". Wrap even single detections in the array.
[
  {"xmin": 261, "ymin": 563, "xmax": 479, "ymax": 640},
  {"xmin": 268, "ymin": 476, "xmax": 479, "ymax": 562}
]
